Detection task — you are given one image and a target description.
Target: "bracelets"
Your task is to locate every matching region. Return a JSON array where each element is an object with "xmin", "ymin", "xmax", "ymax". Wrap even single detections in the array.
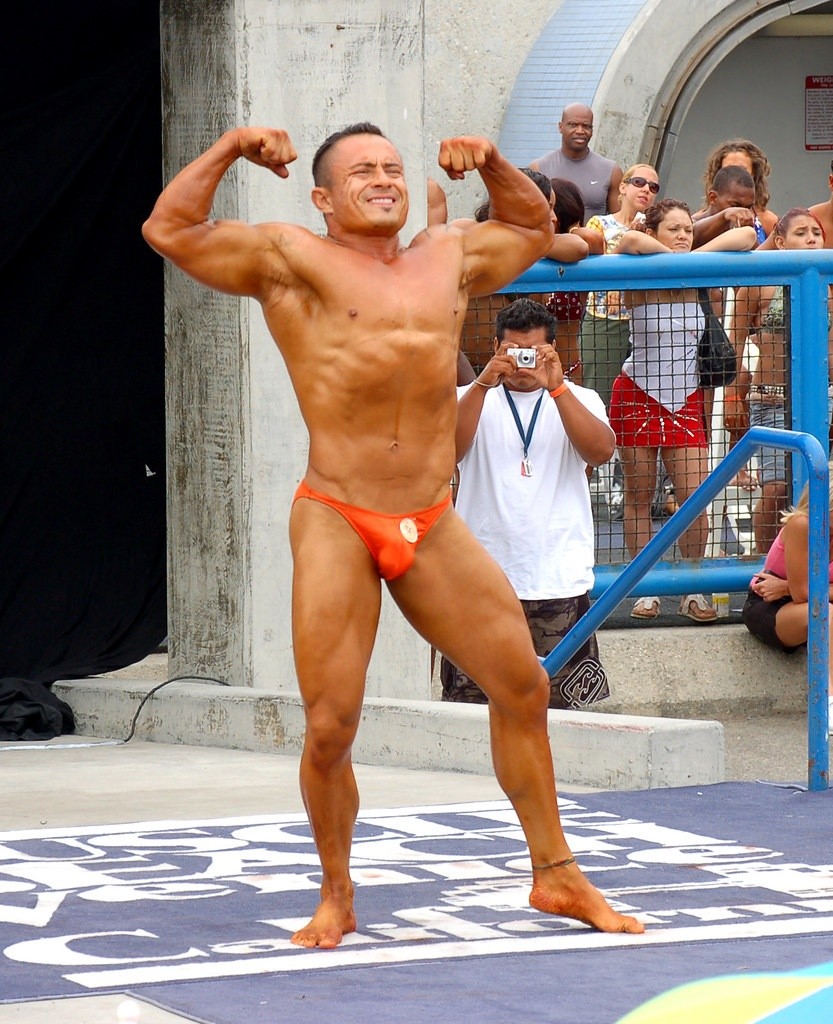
[{"xmin": 550, "ymin": 382, "xmax": 568, "ymax": 398}]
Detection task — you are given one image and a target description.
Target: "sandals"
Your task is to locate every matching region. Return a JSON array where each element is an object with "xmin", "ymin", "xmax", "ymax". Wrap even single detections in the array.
[
  {"xmin": 676, "ymin": 594, "xmax": 717, "ymax": 621},
  {"xmin": 629, "ymin": 596, "xmax": 662, "ymax": 619}
]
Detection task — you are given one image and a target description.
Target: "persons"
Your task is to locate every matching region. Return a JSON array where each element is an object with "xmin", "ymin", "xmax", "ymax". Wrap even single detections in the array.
[
  {"xmin": 458, "ymin": 140, "xmax": 833, "ymax": 622},
  {"xmin": 526, "ymin": 103, "xmax": 623, "ymax": 227},
  {"xmin": 439, "ymin": 297, "xmax": 616, "ymax": 710},
  {"xmin": 142, "ymin": 122, "xmax": 645, "ymax": 950},
  {"xmin": 741, "ymin": 462, "xmax": 833, "ymax": 735}
]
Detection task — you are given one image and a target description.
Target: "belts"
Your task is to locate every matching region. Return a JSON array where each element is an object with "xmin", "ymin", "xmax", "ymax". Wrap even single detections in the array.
[{"xmin": 751, "ymin": 386, "xmax": 784, "ymax": 396}]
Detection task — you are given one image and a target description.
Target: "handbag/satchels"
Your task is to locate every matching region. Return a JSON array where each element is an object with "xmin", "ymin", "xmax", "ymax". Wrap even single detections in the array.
[{"xmin": 697, "ymin": 320, "xmax": 739, "ymax": 388}]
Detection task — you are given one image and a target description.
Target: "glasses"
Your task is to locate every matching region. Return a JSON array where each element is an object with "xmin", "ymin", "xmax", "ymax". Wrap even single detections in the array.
[{"xmin": 623, "ymin": 177, "xmax": 660, "ymax": 195}]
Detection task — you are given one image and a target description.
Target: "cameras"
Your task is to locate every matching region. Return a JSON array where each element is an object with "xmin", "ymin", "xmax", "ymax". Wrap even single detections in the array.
[
  {"xmin": 633, "ymin": 212, "xmax": 646, "ymax": 224},
  {"xmin": 506, "ymin": 348, "xmax": 536, "ymax": 369}
]
